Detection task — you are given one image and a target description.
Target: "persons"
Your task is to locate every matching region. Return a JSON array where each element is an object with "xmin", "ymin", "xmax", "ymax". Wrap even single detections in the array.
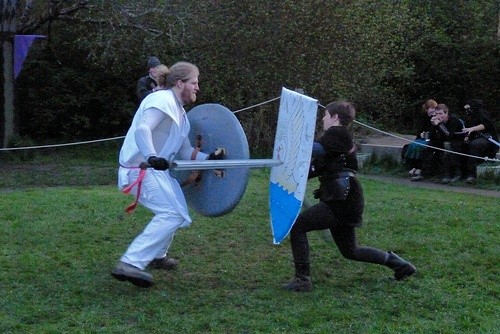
[
  {"xmin": 279, "ymin": 99, "xmax": 416, "ymax": 293},
  {"xmin": 430, "ymin": 104, "xmax": 466, "ymax": 183},
  {"xmin": 404, "ymin": 99, "xmax": 438, "ymax": 179},
  {"xmin": 110, "ymin": 62, "xmax": 225, "ymax": 286},
  {"xmin": 449, "ymin": 103, "xmax": 499, "ymax": 184},
  {"xmin": 136, "ymin": 57, "xmax": 163, "ymax": 102}
]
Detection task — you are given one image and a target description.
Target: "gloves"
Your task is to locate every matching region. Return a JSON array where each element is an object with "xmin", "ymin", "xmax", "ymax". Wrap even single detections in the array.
[
  {"xmin": 146, "ymin": 156, "xmax": 170, "ymax": 171},
  {"xmin": 207, "ymin": 147, "xmax": 226, "ymax": 161}
]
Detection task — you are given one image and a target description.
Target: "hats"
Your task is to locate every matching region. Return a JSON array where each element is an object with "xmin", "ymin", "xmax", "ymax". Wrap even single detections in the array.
[{"xmin": 148, "ymin": 56, "xmax": 161, "ymax": 66}]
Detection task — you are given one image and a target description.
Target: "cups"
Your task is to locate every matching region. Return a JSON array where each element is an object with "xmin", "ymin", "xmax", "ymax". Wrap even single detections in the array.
[{"xmin": 423, "ymin": 131, "xmax": 430, "ymax": 140}]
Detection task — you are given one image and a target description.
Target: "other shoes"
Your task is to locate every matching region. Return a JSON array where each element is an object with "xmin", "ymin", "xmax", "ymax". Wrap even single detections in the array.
[
  {"xmin": 440, "ymin": 174, "xmax": 461, "ymax": 185},
  {"xmin": 465, "ymin": 176, "xmax": 477, "ymax": 188},
  {"xmin": 150, "ymin": 257, "xmax": 180, "ymax": 272},
  {"xmin": 408, "ymin": 166, "xmax": 425, "ymax": 182},
  {"xmin": 109, "ymin": 259, "xmax": 154, "ymax": 286}
]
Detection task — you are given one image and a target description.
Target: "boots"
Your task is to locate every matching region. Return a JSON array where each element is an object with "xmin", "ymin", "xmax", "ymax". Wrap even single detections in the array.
[
  {"xmin": 280, "ymin": 263, "xmax": 314, "ymax": 291},
  {"xmin": 385, "ymin": 250, "xmax": 417, "ymax": 282}
]
